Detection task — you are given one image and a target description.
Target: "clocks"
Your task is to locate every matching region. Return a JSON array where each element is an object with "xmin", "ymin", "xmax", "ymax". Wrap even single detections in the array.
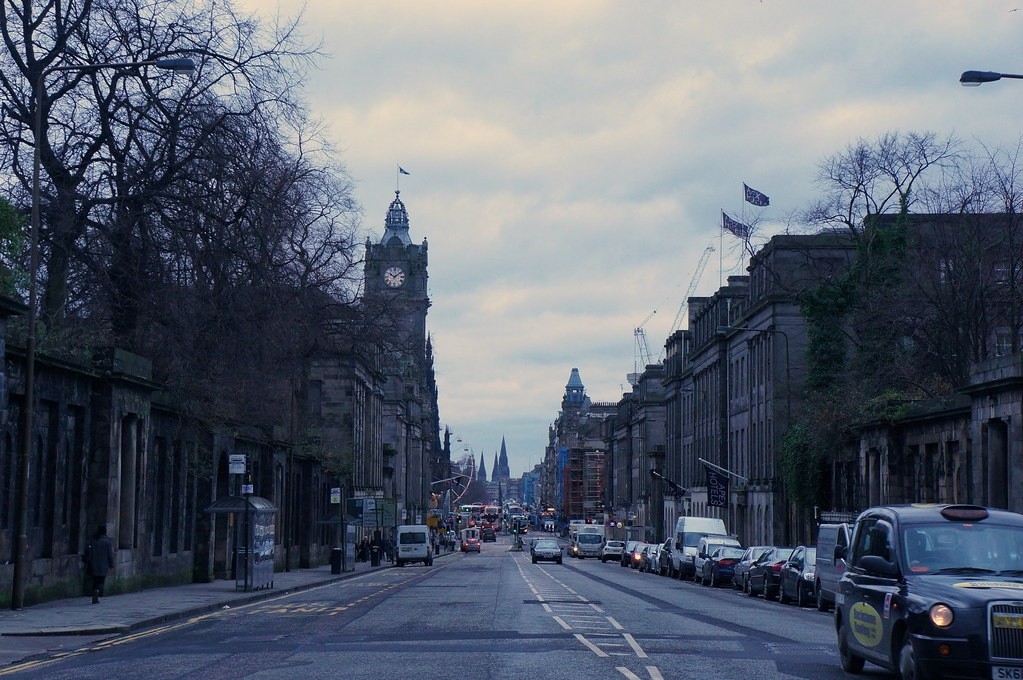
[{"xmin": 383, "ymin": 266, "xmax": 405, "ymax": 289}]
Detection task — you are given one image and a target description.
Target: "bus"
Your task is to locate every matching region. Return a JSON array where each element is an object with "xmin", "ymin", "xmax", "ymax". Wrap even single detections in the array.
[
  {"xmin": 505, "ymin": 502, "xmax": 522, "ymax": 521},
  {"xmin": 459, "ymin": 504, "xmax": 499, "ymax": 515}
]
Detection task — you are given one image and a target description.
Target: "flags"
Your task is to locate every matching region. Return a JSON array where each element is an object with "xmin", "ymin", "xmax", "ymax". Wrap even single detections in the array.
[
  {"xmin": 723, "ymin": 212, "xmax": 748, "ymax": 236},
  {"xmin": 400, "ymin": 167, "xmax": 410, "ymax": 175},
  {"xmin": 745, "ymin": 185, "xmax": 770, "ymax": 206}
]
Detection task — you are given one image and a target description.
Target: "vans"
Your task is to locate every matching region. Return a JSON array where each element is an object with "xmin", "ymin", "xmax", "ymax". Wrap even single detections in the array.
[{"xmin": 694, "ymin": 536, "xmax": 742, "ymax": 586}]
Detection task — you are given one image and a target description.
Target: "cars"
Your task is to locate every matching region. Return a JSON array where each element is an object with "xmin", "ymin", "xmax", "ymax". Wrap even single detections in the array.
[
  {"xmin": 477, "ymin": 514, "xmax": 555, "ymax": 534},
  {"xmin": 731, "ymin": 545, "xmax": 775, "ymax": 591},
  {"xmin": 747, "ymin": 546, "xmax": 795, "ymax": 600},
  {"xmin": 464, "ymin": 538, "xmax": 480, "ymax": 553},
  {"xmin": 701, "ymin": 546, "xmax": 747, "ymax": 587},
  {"xmin": 472, "ymin": 527, "xmax": 496, "ymax": 542},
  {"xmin": 529, "ymin": 539, "xmax": 564, "ymax": 564},
  {"xmin": 834, "ymin": 503, "xmax": 1023, "ymax": 680},
  {"xmin": 630, "ymin": 543, "xmax": 663, "ymax": 575}
]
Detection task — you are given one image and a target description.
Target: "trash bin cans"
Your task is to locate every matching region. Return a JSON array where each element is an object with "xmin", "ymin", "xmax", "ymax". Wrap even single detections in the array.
[
  {"xmin": 371, "ymin": 546, "xmax": 380, "ymax": 566},
  {"xmin": 331, "ymin": 548, "xmax": 343, "ymax": 574}
]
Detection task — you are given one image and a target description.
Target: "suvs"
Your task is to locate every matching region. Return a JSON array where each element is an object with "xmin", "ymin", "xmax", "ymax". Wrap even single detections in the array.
[
  {"xmin": 601, "ymin": 540, "xmax": 626, "ymax": 563},
  {"xmin": 778, "ymin": 545, "xmax": 817, "ymax": 607},
  {"xmin": 658, "ymin": 538, "xmax": 676, "ymax": 577},
  {"xmin": 621, "ymin": 539, "xmax": 649, "ymax": 567}
]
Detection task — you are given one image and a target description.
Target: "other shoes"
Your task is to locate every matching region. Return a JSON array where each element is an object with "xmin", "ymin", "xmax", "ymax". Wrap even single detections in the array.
[{"xmin": 92, "ymin": 600, "xmax": 99, "ymax": 604}]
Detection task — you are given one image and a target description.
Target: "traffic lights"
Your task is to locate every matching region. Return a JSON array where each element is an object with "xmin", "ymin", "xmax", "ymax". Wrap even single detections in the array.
[{"xmin": 445, "ymin": 524, "xmax": 451, "ymax": 535}]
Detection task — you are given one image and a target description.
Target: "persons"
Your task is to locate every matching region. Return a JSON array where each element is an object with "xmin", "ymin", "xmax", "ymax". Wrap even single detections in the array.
[
  {"xmin": 889, "ymin": 530, "xmax": 928, "ymax": 565},
  {"xmin": 359, "ymin": 536, "xmax": 394, "ymax": 563},
  {"xmin": 87, "ymin": 526, "xmax": 114, "ymax": 603}
]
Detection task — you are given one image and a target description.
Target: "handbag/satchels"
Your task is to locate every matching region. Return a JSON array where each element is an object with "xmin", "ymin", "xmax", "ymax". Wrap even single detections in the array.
[{"xmin": 85, "ymin": 540, "xmax": 93, "ymax": 562}]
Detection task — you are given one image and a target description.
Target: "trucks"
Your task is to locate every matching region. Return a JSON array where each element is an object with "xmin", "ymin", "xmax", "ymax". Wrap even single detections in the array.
[
  {"xmin": 813, "ymin": 512, "xmax": 936, "ymax": 612},
  {"xmin": 445, "ymin": 530, "xmax": 456, "ymax": 545},
  {"xmin": 396, "ymin": 525, "xmax": 433, "ymax": 567},
  {"xmin": 569, "ymin": 520, "xmax": 585, "ymax": 539},
  {"xmin": 670, "ymin": 516, "xmax": 727, "ymax": 580},
  {"xmin": 567, "ymin": 524, "xmax": 605, "ymax": 560}
]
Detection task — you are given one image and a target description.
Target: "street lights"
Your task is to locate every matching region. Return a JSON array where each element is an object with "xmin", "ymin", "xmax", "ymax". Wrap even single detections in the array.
[
  {"xmin": 716, "ymin": 325, "xmax": 792, "ymax": 545},
  {"xmin": 10, "ymin": 56, "xmax": 198, "ymax": 612}
]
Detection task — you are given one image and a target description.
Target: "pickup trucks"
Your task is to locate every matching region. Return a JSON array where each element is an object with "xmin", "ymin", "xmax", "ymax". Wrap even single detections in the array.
[{"xmin": 460, "ymin": 528, "xmax": 479, "ymax": 543}]
